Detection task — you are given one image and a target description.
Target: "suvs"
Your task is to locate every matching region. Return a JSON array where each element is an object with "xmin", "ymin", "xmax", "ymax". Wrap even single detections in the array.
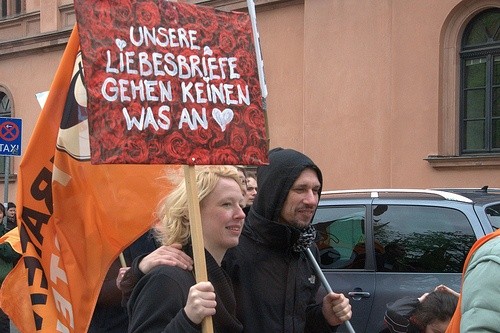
[{"xmin": 308, "ymin": 186, "xmax": 500, "ymax": 329}]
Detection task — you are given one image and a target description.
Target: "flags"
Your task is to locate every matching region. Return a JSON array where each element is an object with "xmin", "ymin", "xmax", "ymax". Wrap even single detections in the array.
[{"xmin": 0, "ymin": 22, "xmax": 207, "ymax": 333}]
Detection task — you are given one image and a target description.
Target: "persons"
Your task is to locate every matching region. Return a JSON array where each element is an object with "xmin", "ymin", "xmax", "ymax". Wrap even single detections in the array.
[
  {"xmin": 383, "ymin": 284, "xmax": 460, "ymax": 333},
  {"xmin": 381, "ymin": 243, "xmax": 411, "ymax": 271},
  {"xmin": 127, "ymin": 164, "xmax": 247, "ymax": 333},
  {"xmin": 121, "ymin": 147, "xmax": 352, "ymax": 333},
  {"xmin": 236, "ymin": 166, "xmax": 258, "ymax": 217},
  {"xmin": 0, "ymin": 202, "xmax": 23, "ymax": 333},
  {"xmin": 86, "ymin": 228, "xmax": 166, "ymax": 333},
  {"xmin": 460, "ymin": 236, "xmax": 500, "ymax": 333}
]
